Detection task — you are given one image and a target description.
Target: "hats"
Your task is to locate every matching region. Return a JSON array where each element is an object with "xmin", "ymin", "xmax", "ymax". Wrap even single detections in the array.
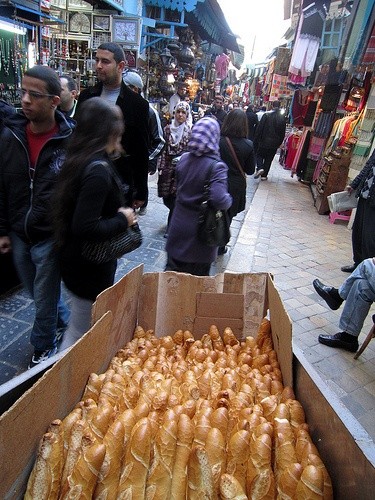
[{"xmin": 123, "ymin": 72, "xmax": 144, "ymax": 90}]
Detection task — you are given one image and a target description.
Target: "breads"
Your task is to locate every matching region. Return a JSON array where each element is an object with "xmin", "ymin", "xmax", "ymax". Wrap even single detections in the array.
[{"xmin": 21, "ymin": 319, "xmax": 333, "ymax": 500}]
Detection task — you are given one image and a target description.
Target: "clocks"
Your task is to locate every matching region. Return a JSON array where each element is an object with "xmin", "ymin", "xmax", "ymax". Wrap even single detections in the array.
[
  {"xmin": 91, "ymin": 13, "xmax": 113, "ymax": 32},
  {"xmin": 111, "ymin": 19, "xmax": 138, "ymax": 43},
  {"xmin": 66, "ymin": 12, "xmax": 91, "ymax": 34}
]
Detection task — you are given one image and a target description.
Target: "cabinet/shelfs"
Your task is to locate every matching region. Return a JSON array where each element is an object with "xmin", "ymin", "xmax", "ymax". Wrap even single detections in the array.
[{"xmin": 313, "ymin": 156, "xmax": 349, "ymax": 215}]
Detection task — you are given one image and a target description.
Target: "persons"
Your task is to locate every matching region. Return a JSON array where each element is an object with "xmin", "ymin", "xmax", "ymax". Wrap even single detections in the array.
[
  {"xmin": 0, "ymin": 64, "xmax": 70, "ymax": 371},
  {"xmin": 123, "ymin": 71, "xmax": 165, "ymax": 216},
  {"xmin": 340, "ymin": 149, "xmax": 374, "ymax": 271},
  {"xmin": 161, "ymin": 85, "xmax": 286, "ymax": 181},
  {"xmin": 58, "ymin": 75, "xmax": 79, "ymax": 120},
  {"xmin": 78, "ymin": 42, "xmax": 150, "ymax": 210},
  {"xmin": 163, "ymin": 118, "xmax": 231, "ymax": 276},
  {"xmin": 0, "ymin": 70, "xmax": 16, "ymax": 128},
  {"xmin": 314, "ymin": 256, "xmax": 375, "ymax": 352},
  {"xmin": 157, "ymin": 101, "xmax": 194, "ymax": 239},
  {"xmin": 218, "ymin": 109, "xmax": 255, "ymax": 254},
  {"xmin": 59, "ymin": 97, "xmax": 139, "ymax": 352}
]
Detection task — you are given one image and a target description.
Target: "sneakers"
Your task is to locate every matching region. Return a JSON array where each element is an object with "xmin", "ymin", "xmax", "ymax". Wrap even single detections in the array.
[
  {"xmin": 56, "ymin": 310, "xmax": 72, "ymax": 349},
  {"xmin": 28, "ymin": 331, "xmax": 58, "ymax": 371}
]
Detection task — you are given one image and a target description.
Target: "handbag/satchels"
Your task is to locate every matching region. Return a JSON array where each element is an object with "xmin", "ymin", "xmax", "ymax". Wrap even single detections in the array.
[
  {"xmin": 196, "ymin": 160, "xmax": 231, "ymax": 250},
  {"xmin": 71, "ymin": 160, "xmax": 143, "ymax": 265}
]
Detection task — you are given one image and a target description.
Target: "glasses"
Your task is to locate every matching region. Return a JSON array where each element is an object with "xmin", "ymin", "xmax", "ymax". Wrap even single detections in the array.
[{"xmin": 17, "ymin": 88, "xmax": 56, "ymax": 98}]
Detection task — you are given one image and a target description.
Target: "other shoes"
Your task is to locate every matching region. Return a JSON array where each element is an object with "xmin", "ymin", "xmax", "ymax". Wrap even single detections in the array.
[
  {"xmin": 218, "ymin": 246, "xmax": 227, "ymax": 255},
  {"xmin": 254, "ymin": 169, "xmax": 264, "ymax": 179},
  {"xmin": 341, "ymin": 265, "xmax": 356, "ymax": 273},
  {"xmin": 261, "ymin": 177, "xmax": 267, "ymax": 181},
  {"xmin": 164, "ymin": 224, "xmax": 168, "ymax": 238}
]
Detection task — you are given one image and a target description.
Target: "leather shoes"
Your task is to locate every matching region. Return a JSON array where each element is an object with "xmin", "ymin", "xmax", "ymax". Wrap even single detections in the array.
[
  {"xmin": 318, "ymin": 333, "xmax": 358, "ymax": 353},
  {"xmin": 313, "ymin": 279, "xmax": 342, "ymax": 310}
]
